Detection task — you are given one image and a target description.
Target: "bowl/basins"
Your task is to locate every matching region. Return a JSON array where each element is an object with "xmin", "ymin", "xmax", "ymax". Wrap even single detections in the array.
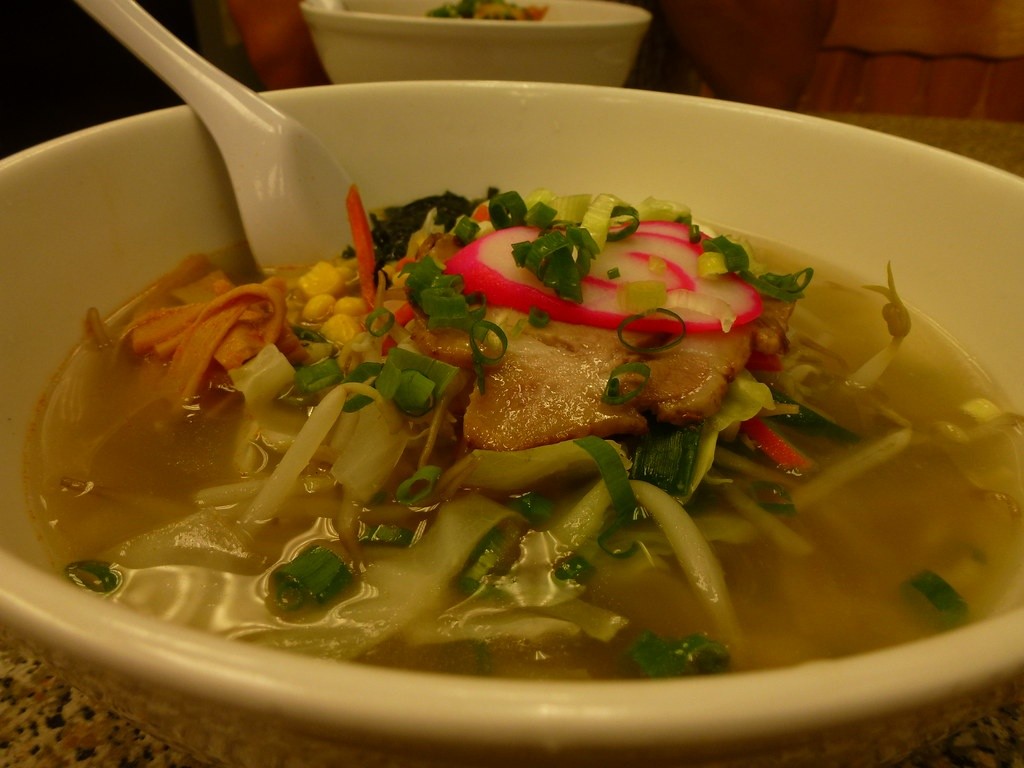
[
  {"xmin": 300, "ymin": 0, "xmax": 653, "ymax": 89},
  {"xmin": 0, "ymin": 78, "xmax": 1024, "ymax": 768}
]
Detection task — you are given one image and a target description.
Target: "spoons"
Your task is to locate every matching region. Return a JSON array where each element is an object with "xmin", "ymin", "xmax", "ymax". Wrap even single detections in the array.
[{"xmin": 70, "ymin": 0, "xmax": 354, "ymax": 279}]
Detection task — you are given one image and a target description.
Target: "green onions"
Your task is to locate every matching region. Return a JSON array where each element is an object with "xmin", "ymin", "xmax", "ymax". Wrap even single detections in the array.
[{"xmin": 75, "ymin": 188, "xmax": 970, "ymax": 680}]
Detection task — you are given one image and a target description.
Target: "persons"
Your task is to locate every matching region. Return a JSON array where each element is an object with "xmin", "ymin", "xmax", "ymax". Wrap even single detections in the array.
[{"xmin": 223, "ymin": 0, "xmax": 834, "ymax": 113}]
